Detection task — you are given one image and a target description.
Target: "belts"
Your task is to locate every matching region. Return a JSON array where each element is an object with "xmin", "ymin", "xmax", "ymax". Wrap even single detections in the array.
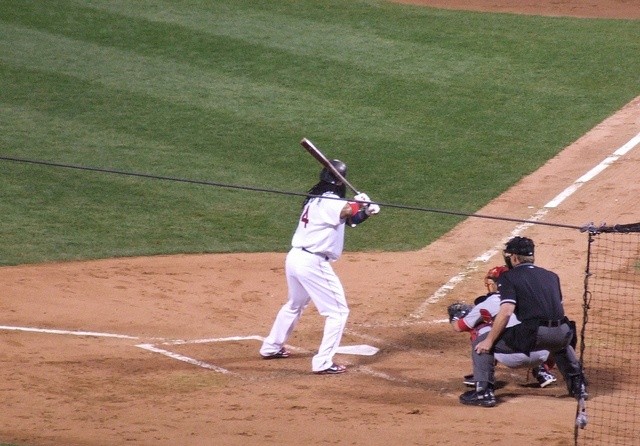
[
  {"xmin": 302, "ymin": 247, "xmax": 329, "ymax": 260},
  {"xmin": 539, "ymin": 316, "xmax": 569, "ymax": 328}
]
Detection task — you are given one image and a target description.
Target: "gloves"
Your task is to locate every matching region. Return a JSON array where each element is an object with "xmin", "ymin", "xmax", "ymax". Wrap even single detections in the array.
[
  {"xmin": 353, "ymin": 192, "xmax": 372, "ymax": 206},
  {"xmin": 364, "ymin": 203, "xmax": 380, "ymax": 217}
]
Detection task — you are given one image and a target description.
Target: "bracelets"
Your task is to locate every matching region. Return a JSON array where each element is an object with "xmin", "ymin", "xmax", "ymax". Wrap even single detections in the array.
[{"xmin": 348, "ymin": 201, "xmax": 360, "ymax": 216}]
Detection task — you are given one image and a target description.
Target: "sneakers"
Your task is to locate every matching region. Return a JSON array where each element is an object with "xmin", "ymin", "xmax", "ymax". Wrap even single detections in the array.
[
  {"xmin": 569, "ymin": 382, "xmax": 589, "ymax": 401},
  {"xmin": 462, "ymin": 376, "xmax": 495, "ymax": 385},
  {"xmin": 537, "ymin": 371, "xmax": 557, "ymax": 388},
  {"xmin": 459, "ymin": 388, "xmax": 496, "ymax": 408},
  {"xmin": 313, "ymin": 363, "xmax": 346, "ymax": 374},
  {"xmin": 259, "ymin": 347, "xmax": 289, "ymax": 358}
]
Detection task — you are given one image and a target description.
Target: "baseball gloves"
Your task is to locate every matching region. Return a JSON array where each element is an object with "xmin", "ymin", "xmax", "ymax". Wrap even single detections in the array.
[{"xmin": 447, "ymin": 302, "xmax": 473, "ymax": 319}]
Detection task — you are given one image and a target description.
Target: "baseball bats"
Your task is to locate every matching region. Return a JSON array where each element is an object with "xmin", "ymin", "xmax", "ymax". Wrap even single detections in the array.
[{"xmin": 300, "ymin": 137, "xmax": 380, "ymax": 215}]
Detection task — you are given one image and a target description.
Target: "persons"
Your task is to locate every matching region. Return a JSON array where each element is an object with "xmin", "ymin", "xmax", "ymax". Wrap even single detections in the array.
[
  {"xmin": 448, "ymin": 265, "xmax": 557, "ymax": 388},
  {"xmin": 259, "ymin": 159, "xmax": 381, "ymax": 375},
  {"xmin": 459, "ymin": 236, "xmax": 587, "ymax": 408}
]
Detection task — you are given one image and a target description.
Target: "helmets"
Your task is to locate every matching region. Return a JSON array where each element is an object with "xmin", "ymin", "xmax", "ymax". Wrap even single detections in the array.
[
  {"xmin": 484, "ymin": 265, "xmax": 510, "ymax": 295},
  {"xmin": 502, "ymin": 236, "xmax": 534, "ymax": 269},
  {"xmin": 319, "ymin": 159, "xmax": 347, "ymax": 186}
]
